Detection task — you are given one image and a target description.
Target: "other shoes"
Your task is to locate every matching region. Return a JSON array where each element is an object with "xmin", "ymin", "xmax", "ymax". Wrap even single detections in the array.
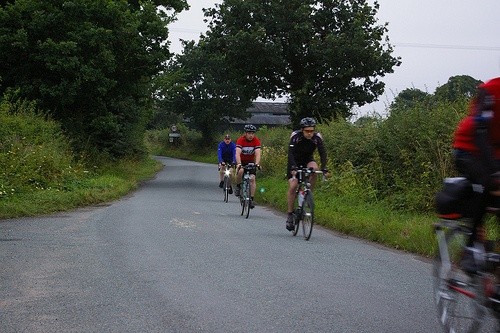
[
  {"xmin": 228, "ymin": 186, "xmax": 233, "ymax": 194},
  {"xmin": 250, "ymin": 199, "xmax": 255, "ymax": 209},
  {"xmin": 308, "ymin": 202, "xmax": 316, "ymax": 209},
  {"xmin": 286, "ymin": 220, "xmax": 293, "ymax": 231},
  {"xmin": 234, "ymin": 187, "xmax": 240, "ymax": 197},
  {"xmin": 219, "ymin": 181, "xmax": 224, "ymax": 188}
]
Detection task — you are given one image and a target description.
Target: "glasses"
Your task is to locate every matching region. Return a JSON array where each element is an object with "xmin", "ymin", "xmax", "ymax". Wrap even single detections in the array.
[{"xmin": 302, "ymin": 129, "xmax": 314, "ymax": 133}]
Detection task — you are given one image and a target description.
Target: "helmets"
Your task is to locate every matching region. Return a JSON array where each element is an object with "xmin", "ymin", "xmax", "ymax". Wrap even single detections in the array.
[
  {"xmin": 300, "ymin": 117, "xmax": 316, "ymax": 127},
  {"xmin": 244, "ymin": 125, "xmax": 257, "ymax": 132},
  {"xmin": 225, "ymin": 134, "xmax": 231, "ymax": 139}
]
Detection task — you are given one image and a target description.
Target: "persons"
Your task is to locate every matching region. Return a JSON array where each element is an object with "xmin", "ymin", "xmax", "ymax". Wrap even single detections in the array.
[
  {"xmin": 452, "ymin": 76, "xmax": 500, "ymax": 274},
  {"xmin": 286, "ymin": 117, "xmax": 329, "ymax": 230},
  {"xmin": 234, "ymin": 124, "xmax": 261, "ymax": 208},
  {"xmin": 217, "ymin": 134, "xmax": 236, "ymax": 194}
]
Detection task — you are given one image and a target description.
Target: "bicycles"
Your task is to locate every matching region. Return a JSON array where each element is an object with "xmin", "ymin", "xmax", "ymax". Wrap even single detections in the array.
[
  {"xmin": 238, "ymin": 162, "xmax": 262, "ymax": 220},
  {"xmin": 431, "ymin": 176, "xmax": 500, "ymax": 333},
  {"xmin": 217, "ymin": 163, "xmax": 239, "ymax": 203},
  {"xmin": 285, "ymin": 165, "xmax": 328, "ymax": 240}
]
